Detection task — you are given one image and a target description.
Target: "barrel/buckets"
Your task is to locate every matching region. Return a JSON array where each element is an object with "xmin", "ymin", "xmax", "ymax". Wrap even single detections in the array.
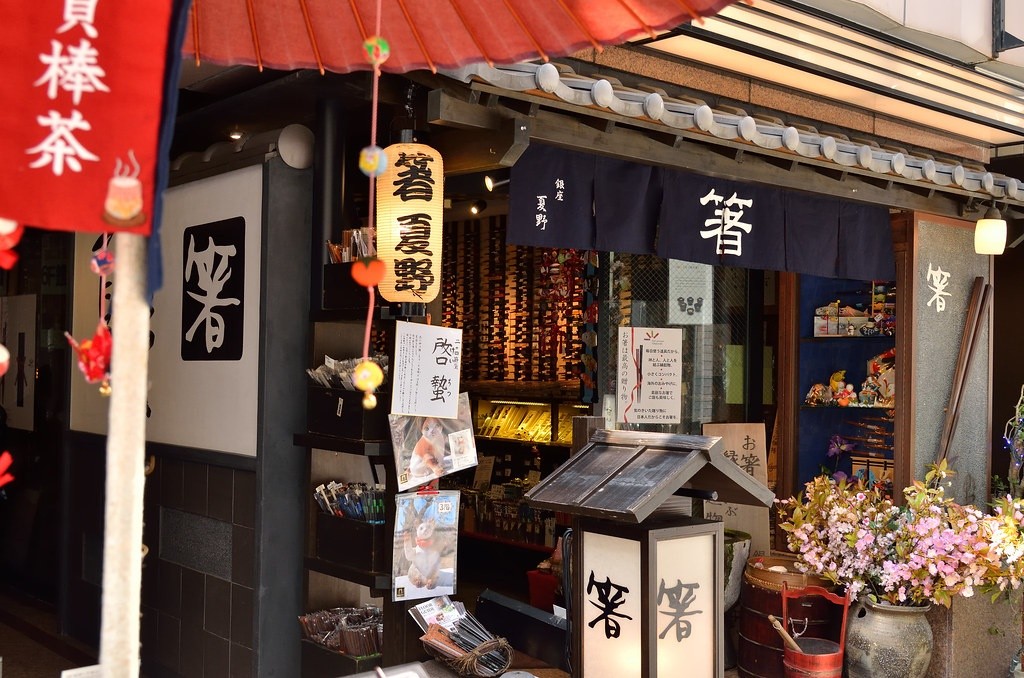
[
  {"xmin": 780, "ymin": 582, "xmax": 850, "ymax": 678},
  {"xmin": 738, "ymin": 556, "xmax": 839, "ymax": 678}
]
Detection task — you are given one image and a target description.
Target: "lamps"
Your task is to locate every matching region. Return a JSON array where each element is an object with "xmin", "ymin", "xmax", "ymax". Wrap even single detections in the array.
[{"xmin": 974, "ymin": 195, "xmax": 1008, "ymax": 257}]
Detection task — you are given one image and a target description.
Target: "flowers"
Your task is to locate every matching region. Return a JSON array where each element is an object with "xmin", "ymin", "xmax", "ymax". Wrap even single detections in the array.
[{"xmin": 774, "ymin": 458, "xmax": 1024, "ymax": 606}]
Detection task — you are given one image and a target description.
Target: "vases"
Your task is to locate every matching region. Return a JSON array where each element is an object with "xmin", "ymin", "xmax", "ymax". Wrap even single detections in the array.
[{"xmin": 844, "ymin": 595, "xmax": 935, "ymax": 678}]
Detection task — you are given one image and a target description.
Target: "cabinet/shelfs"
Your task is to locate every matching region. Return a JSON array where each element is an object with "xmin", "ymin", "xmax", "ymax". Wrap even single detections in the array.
[
  {"xmin": 459, "ymin": 380, "xmax": 588, "ymax": 554},
  {"xmin": 291, "ymin": 255, "xmax": 434, "ymax": 678},
  {"xmin": 776, "ymin": 207, "xmax": 917, "ymax": 554}
]
